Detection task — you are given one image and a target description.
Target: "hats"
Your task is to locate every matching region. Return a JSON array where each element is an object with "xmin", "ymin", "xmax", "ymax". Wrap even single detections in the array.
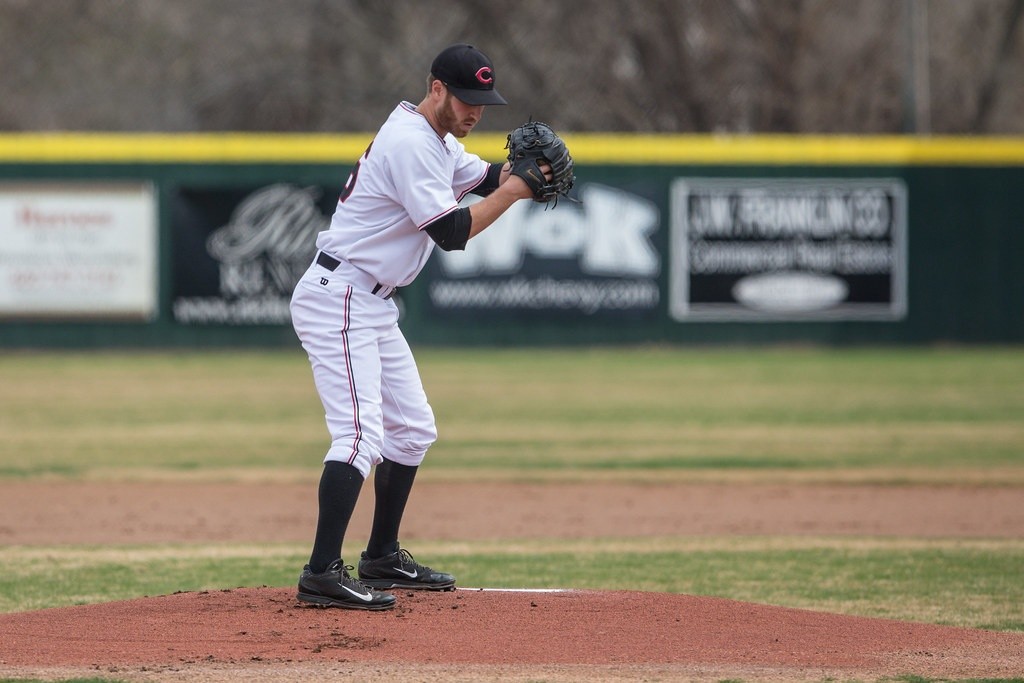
[{"xmin": 431, "ymin": 44, "xmax": 508, "ymax": 105}]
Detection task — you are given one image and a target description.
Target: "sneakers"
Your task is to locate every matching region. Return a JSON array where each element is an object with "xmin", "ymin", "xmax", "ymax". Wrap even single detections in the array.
[
  {"xmin": 357, "ymin": 542, "xmax": 456, "ymax": 592},
  {"xmin": 297, "ymin": 557, "xmax": 396, "ymax": 611}
]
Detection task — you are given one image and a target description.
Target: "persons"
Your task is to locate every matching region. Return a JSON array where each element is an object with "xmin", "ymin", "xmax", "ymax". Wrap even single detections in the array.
[{"xmin": 289, "ymin": 44, "xmax": 577, "ymax": 612}]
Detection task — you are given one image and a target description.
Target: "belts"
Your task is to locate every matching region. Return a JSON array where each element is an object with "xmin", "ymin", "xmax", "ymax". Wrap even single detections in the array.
[{"xmin": 316, "ymin": 251, "xmax": 396, "ymax": 300}]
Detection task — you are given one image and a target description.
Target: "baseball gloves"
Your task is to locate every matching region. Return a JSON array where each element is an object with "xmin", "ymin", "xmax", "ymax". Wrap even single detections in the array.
[{"xmin": 507, "ymin": 121, "xmax": 575, "ymax": 204}]
